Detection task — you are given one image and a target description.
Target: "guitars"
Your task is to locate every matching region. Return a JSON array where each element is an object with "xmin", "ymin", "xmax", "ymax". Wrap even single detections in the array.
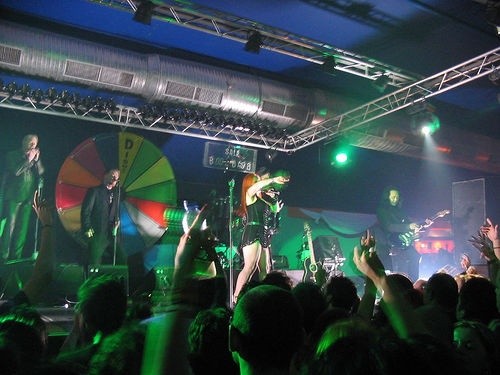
[
  {"xmin": 302, "ymin": 221, "xmax": 327, "ymax": 287},
  {"xmin": 386, "ymin": 208, "xmax": 451, "ymax": 250}
]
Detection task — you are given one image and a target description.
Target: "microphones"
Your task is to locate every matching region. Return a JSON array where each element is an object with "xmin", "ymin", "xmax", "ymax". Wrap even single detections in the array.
[{"xmin": 262, "ymin": 190, "xmax": 280, "ymax": 195}]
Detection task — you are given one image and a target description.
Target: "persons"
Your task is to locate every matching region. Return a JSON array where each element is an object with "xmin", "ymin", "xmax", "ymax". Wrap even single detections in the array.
[
  {"xmin": 0, "ymin": 218, "xmax": 500, "ymax": 375},
  {"xmin": 80, "ymin": 169, "xmax": 126, "ymax": 279},
  {"xmin": 0, "ymin": 133, "xmax": 44, "ymax": 260},
  {"xmin": 376, "ymin": 186, "xmax": 426, "ymax": 282},
  {"xmin": 233, "ymin": 167, "xmax": 290, "ymax": 305}
]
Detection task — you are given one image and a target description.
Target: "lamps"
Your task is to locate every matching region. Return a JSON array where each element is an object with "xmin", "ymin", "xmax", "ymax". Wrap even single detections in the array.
[
  {"xmin": 410, "ymin": 113, "xmax": 440, "ymax": 136},
  {"xmin": 318, "ymin": 56, "xmax": 336, "ymax": 77},
  {"xmin": 371, "ymin": 71, "xmax": 389, "ymax": 92},
  {"xmin": 242, "ymin": 31, "xmax": 262, "ymax": 55},
  {"xmin": 132, "ymin": 0, "xmax": 156, "ymax": 25}
]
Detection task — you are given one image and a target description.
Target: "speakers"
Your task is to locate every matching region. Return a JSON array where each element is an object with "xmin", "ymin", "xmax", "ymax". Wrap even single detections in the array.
[
  {"xmin": 313, "ymin": 236, "xmax": 343, "ymax": 264},
  {"xmin": 452, "ymin": 177, "xmax": 500, "ymax": 283}
]
